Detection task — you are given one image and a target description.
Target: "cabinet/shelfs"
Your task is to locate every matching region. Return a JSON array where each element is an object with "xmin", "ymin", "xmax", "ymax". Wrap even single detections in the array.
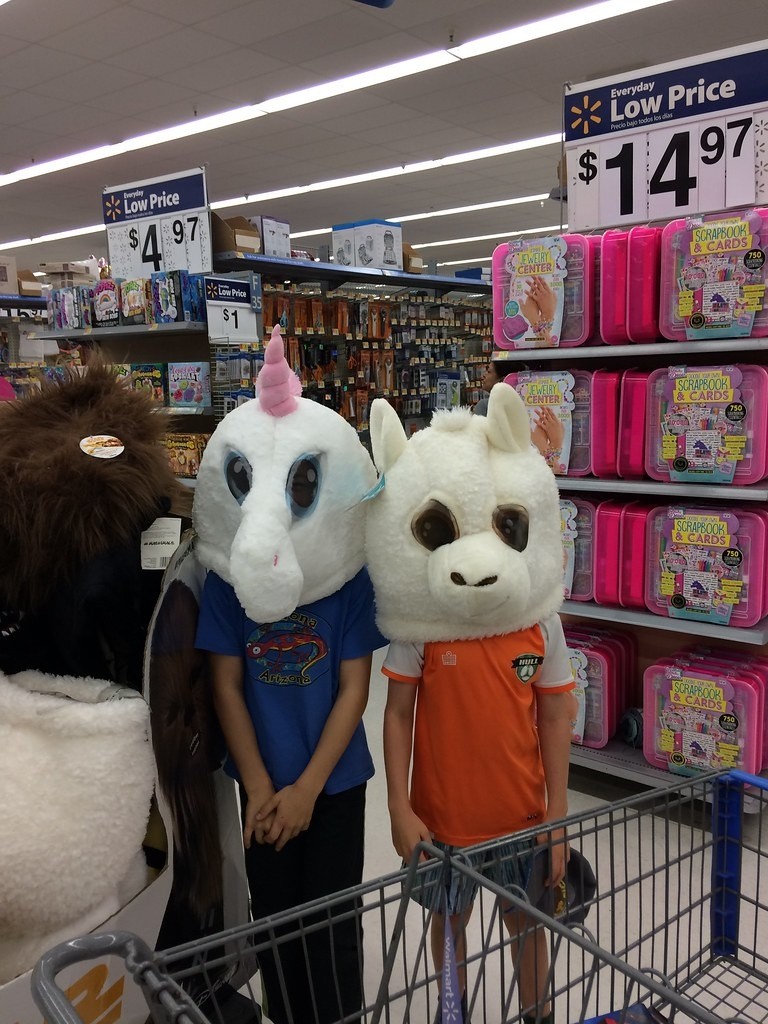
[{"xmin": 4, "ymin": 254, "xmax": 768, "ymax": 814}]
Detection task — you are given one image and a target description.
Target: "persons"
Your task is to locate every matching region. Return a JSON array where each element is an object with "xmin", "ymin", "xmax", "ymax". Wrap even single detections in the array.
[
  {"xmin": 517, "ymin": 272, "xmax": 557, "ymax": 349},
  {"xmin": 199, "ymin": 397, "xmax": 385, "ymax": 1024},
  {"xmin": 529, "ymin": 405, "xmax": 565, "ymax": 465},
  {"xmin": 380, "ymin": 416, "xmax": 576, "ymax": 1023},
  {"xmin": 473, "ymin": 360, "xmax": 526, "ymax": 417}
]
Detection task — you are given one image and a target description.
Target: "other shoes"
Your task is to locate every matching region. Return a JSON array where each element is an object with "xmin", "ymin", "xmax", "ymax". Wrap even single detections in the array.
[
  {"xmin": 521, "ymin": 1010, "xmax": 553, "ymax": 1024},
  {"xmin": 433, "ymin": 986, "xmax": 468, "ymax": 1024}
]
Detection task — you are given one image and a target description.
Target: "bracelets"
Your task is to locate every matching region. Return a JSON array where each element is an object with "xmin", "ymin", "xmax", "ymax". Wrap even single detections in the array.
[
  {"xmin": 542, "ymin": 318, "xmax": 554, "ymax": 329},
  {"xmin": 551, "ymin": 447, "xmax": 562, "ymax": 460},
  {"xmin": 531, "ymin": 320, "xmax": 544, "ymax": 333},
  {"xmin": 541, "ymin": 448, "xmax": 554, "ymax": 462}
]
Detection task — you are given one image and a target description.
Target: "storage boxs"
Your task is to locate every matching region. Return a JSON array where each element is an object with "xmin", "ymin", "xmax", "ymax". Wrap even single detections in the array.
[
  {"xmin": 207, "ymin": 210, "xmax": 261, "ymax": 254},
  {"xmin": 330, "ymin": 219, "xmax": 405, "ymax": 271},
  {"xmin": 15, "ymin": 269, "xmax": 43, "ymax": 297},
  {"xmin": 248, "ymin": 214, "xmax": 292, "ymax": 259}
]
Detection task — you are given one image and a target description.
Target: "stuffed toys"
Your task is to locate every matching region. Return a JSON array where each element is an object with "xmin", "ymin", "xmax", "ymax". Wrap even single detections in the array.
[{"xmin": 0, "ymin": 351, "xmax": 221, "ymax": 986}]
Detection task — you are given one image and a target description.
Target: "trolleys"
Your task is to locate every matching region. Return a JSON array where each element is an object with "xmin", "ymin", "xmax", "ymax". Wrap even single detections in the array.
[{"xmin": 28, "ymin": 771, "xmax": 766, "ymax": 1024}]
